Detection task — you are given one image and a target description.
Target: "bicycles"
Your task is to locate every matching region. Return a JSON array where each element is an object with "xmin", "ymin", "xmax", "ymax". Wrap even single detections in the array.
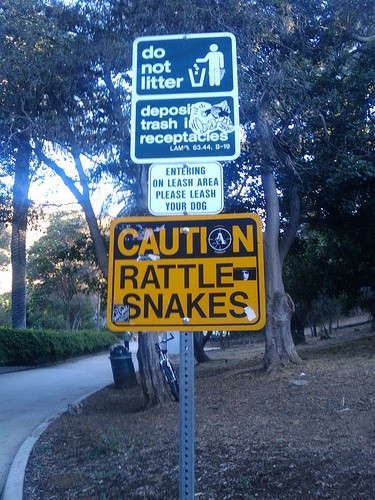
[{"xmin": 155, "ymin": 333, "xmax": 179, "ymax": 402}]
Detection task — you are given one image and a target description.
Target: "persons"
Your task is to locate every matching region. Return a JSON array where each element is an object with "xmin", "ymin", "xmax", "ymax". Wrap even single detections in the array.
[{"xmin": 123, "ymin": 330, "xmax": 132, "ymax": 351}]
